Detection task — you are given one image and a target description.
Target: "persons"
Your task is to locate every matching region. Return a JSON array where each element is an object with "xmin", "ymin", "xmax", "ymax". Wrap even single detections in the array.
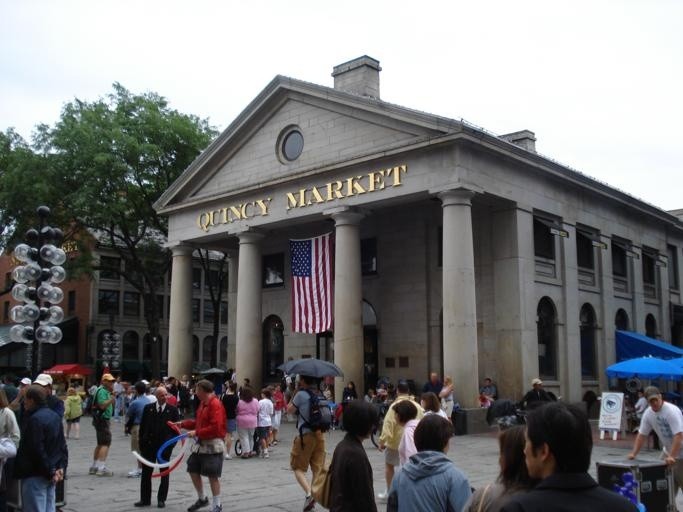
[{"xmin": 0, "ymin": 357, "xmax": 683, "ymax": 512}]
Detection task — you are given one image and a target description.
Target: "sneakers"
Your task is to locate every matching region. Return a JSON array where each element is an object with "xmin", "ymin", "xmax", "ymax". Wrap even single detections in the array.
[
  {"xmin": 209, "ymin": 504, "xmax": 222, "ymax": 512},
  {"xmin": 128, "ymin": 470, "xmax": 139, "ymax": 478},
  {"xmin": 303, "ymin": 495, "xmax": 315, "ymax": 512},
  {"xmin": 95, "ymin": 469, "xmax": 113, "ymax": 477},
  {"xmin": 377, "ymin": 490, "xmax": 388, "ymax": 500},
  {"xmin": 259, "ymin": 449, "xmax": 269, "ymax": 458},
  {"xmin": 225, "ymin": 454, "xmax": 232, "ymax": 459},
  {"xmin": 272, "ymin": 440, "xmax": 278, "ymax": 445},
  {"xmin": 188, "ymin": 496, "xmax": 208, "ymax": 512},
  {"xmin": 89, "ymin": 467, "xmax": 98, "ymax": 475}
]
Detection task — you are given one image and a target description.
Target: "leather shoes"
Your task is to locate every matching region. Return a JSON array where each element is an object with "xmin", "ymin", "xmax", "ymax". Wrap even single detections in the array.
[
  {"xmin": 135, "ymin": 500, "xmax": 150, "ymax": 507},
  {"xmin": 158, "ymin": 499, "xmax": 165, "ymax": 508}
]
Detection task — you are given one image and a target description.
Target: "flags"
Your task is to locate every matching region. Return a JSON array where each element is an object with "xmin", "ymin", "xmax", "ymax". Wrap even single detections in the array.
[{"xmin": 290, "ymin": 232, "xmax": 333, "ymax": 334}]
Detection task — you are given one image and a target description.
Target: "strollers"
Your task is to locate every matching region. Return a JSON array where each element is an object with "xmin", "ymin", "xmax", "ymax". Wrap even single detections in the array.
[{"xmin": 234, "ymin": 422, "xmax": 265, "ymax": 457}]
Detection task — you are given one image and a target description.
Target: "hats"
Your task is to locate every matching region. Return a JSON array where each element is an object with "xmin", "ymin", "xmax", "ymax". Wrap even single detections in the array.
[
  {"xmin": 19, "ymin": 377, "xmax": 31, "ymax": 386},
  {"xmin": 532, "ymin": 378, "xmax": 543, "ymax": 386},
  {"xmin": 102, "ymin": 373, "xmax": 115, "ymax": 381},
  {"xmin": 141, "ymin": 379, "xmax": 150, "ymax": 385},
  {"xmin": 32, "ymin": 374, "xmax": 53, "ymax": 387},
  {"xmin": 68, "ymin": 388, "xmax": 75, "ymax": 393},
  {"xmin": 644, "ymin": 386, "xmax": 661, "ymax": 401}
]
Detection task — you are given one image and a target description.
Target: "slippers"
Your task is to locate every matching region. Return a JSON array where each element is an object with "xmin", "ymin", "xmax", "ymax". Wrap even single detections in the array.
[{"xmin": 241, "ymin": 456, "xmax": 248, "ymax": 459}]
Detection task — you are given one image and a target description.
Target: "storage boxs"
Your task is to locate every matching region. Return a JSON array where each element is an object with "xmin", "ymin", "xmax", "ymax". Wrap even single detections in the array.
[{"xmin": 596, "ymin": 458, "xmax": 668, "ymax": 512}]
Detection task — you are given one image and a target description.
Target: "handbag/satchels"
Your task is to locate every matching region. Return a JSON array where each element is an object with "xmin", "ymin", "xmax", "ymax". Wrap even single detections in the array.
[
  {"xmin": 311, "ymin": 466, "xmax": 332, "ymax": 509},
  {"xmin": 92, "ymin": 404, "xmax": 103, "ymax": 417},
  {"xmin": 125, "ymin": 417, "xmax": 134, "ymax": 434},
  {"xmin": 387, "ymin": 491, "xmax": 398, "ymax": 512}
]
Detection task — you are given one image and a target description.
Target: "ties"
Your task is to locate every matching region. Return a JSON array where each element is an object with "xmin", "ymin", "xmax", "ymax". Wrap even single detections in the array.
[{"xmin": 157, "ymin": 406, "xmax": 162, "ymax": 417}]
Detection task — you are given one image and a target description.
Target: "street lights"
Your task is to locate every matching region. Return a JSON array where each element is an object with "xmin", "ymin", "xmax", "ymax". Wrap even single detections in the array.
[
  {"xmin": 98, "ymin": 329, "xmax": 119, "ymax": 378},
  {"xmin": 7, "ymin": 205, "xmax": 67, "ymax": 381}
]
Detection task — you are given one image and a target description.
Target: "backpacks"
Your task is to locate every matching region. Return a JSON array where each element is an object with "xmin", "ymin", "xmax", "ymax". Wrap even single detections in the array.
[{"xmin": 308, "ymin": 393, "xmax": 331, "ymax": 433}]
